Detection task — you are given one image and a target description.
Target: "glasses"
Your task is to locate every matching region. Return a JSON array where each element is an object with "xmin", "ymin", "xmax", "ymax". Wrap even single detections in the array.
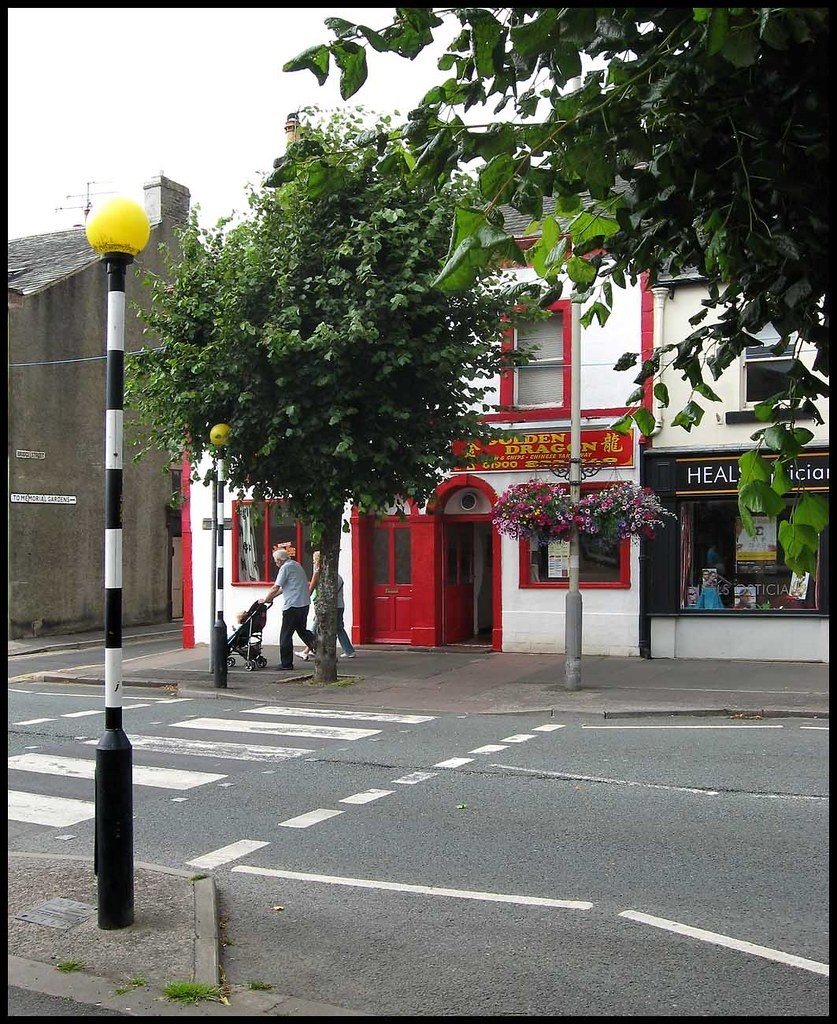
[
  {"xmin": 688, "ymin": 592, "xmax": 696, "ymax": 596},
  {"xmin": 704, "ymin": 574, "xmax": 709, "ymax": 575}
]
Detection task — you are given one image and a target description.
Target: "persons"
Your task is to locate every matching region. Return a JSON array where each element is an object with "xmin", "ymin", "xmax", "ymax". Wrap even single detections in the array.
[
  {"xmin": 294, "ymin": 549, "xmax": 350, "ymax": 661},
  {"xmin": 737, "ymin": 589, "xmax": 752, "ymax": 608},
  {"xmin": 703, "ymin": 570, "xmax": 717, "ymax": 588},
  {"xmin": 791, "ymin": 572, "xmax": 806, "ymax": 598},
  {"xmin": 263, "ymin": 549, "xmax": 316, "ymax": 670},
  {"xmin": 688, "ymin": 588, "xmax": 698, "ymax": 604},
  {"xmin": 227, "ymin": 611, "xmax": 248, "ymax": 641}
]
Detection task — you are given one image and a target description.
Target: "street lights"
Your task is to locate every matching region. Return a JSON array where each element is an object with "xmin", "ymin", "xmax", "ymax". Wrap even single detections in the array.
[
  {"xmin": 84, "ymin": 192, "xmax": 150, "ymax": 931},
  {"xmin": 210, "ymin": 424, "xmax": 232, "ymax": 689}
]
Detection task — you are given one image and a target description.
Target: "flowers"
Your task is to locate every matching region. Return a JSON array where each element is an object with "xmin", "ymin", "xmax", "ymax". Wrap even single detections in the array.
[
  {"xmin": 483, "ymin": 477, "xmax": 577, "ymax": 543},
  {"xmin": 575, "ymin": 480, "xmax": 679, "ymax": 544}
]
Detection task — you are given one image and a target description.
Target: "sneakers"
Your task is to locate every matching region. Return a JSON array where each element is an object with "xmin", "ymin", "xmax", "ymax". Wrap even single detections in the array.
[
  {"xmin": 295, "ymin": 652, "xmax": 308, "ymax": 660},
  {"xmin": 309, "ymin": 650, "xmax": 316, "ymax": 656},
  {"xmin": 340, "ymin": 651, "xmax": 355, "ymax": 657}
]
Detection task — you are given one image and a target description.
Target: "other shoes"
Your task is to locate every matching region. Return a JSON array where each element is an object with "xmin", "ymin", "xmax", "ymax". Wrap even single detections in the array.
[{"xmin": 277, "ymin": 663, "xmax": 293, "ymax": 670}]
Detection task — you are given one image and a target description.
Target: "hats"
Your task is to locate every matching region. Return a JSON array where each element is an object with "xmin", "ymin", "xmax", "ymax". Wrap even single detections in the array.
[{"xmin": 740, "ymin": 589, "xmax": 755, "ymax": 597}]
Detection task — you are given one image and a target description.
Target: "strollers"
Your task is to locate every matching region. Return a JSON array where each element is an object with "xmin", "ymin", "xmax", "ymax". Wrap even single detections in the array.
[{"xmin": 228, "ymin": 598, "xmax": 273, "ymax": 671}]
